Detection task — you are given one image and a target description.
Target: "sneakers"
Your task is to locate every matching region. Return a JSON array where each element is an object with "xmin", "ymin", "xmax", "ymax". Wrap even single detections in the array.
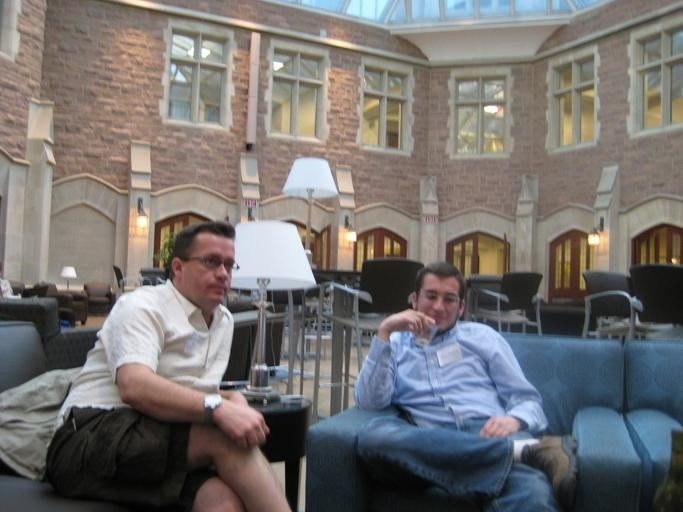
[{"xmin": 522, "ymin": 432, "xmax": 578, "ymax": 506}]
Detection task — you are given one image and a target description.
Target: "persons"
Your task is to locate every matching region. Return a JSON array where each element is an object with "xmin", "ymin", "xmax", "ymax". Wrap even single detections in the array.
[
  {"xmin": 44, "ymin": 219, "xmax": 290, "ymax": 512},
  {"xmin": 351, "ymin": 263, "xmax": 580, "ymax": 512}
]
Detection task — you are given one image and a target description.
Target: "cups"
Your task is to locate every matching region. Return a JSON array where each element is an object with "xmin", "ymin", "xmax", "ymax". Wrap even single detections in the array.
[{"xmin": 410, "ymin": 323, "xmax": 438, "ymax": 350}]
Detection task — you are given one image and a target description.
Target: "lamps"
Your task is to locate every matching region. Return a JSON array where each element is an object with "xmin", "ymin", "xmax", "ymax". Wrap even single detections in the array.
[
  {"xmin": 346, "ymin": 217, "xmax": 357, "ymax": 241},
  {"xmin": 230, "ymin": 221, "xmax": 318, "ymax": 407},
  {"xmin": 137, "ymin": 201, "xmax": 150, "ymax": 230},
  {"xmin": 587, "ymin": 218, "xmax": 604, "ymax": 245},
  {"xmin": 283, "ymin": 158, "xmax": 338, "ymax": 269}
]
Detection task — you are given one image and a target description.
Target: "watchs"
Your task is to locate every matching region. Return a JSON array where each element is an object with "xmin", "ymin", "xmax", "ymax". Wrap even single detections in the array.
[{"xmin": 202, "ymin": 393, "xmax": 222, "ymax": 417}]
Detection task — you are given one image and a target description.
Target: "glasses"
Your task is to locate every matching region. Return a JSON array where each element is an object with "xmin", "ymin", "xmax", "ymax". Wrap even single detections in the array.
[
  {"xmin": 418, "ymin": 290, "xmax": 463, "ymax": 306},
  {"xmin": 183, "ymin": 256, "xmax": 238, "ymax": 271}
]
